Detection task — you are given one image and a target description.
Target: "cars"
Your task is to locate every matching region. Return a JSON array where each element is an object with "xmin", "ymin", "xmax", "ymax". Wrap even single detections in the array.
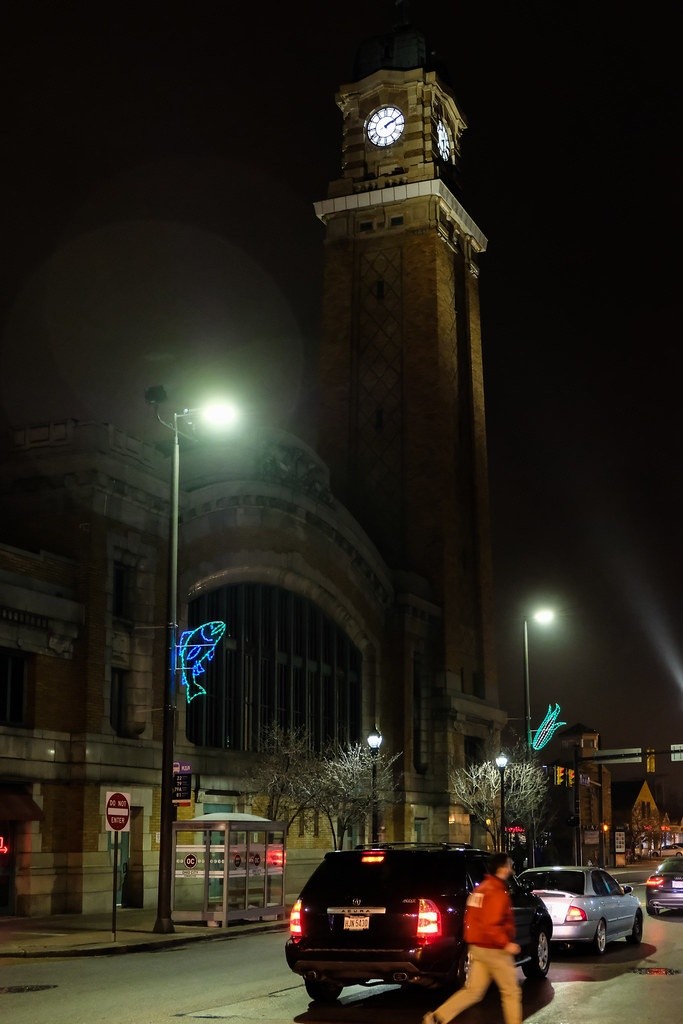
[
  {"xmin": 647, "ymin": 857, "xmax": 683, "ymax": 921},
  {"xmin": 519, "ymin": 864, "xmax": 643, "ymax": 958}
]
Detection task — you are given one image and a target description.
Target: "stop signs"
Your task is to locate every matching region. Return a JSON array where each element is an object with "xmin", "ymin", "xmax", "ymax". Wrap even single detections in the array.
[{"xmin": 103, "ymin": 789, "xmax": 132, "ymax": 835}]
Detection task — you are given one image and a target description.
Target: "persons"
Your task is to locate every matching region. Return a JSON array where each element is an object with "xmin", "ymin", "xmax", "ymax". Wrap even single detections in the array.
[{"xmin": 420, "ymin": 851, "xmax": 527, "ymax": 1024}]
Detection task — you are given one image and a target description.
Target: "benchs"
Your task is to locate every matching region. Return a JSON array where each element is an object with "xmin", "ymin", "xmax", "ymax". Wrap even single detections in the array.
[{"xmin": 199, "ymin": 895, "xmax": 264, "ymax": 928}]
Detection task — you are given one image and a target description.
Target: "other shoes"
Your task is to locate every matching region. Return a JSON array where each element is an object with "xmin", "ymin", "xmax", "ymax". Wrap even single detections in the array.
[{"xmin": 423, "ymin": 1012, "xmax": 440, "ymax": 1024}]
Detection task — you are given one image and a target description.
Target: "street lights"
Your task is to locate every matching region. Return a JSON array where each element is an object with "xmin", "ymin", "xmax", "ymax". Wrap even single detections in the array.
[
  {"xmin": 155, "ymin": 393, "xmax": 239, "ymax": 934},
  {"xmin": 521, "ymin": 596, "xmax": 560, "ymax": 877}
]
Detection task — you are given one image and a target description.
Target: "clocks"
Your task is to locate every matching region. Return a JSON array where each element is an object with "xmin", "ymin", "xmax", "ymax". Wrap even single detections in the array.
[
  {"xmin": 435, "ymin": 119, "xmax": 450, "ymax": 161},
  {"xmin": 365, "ymin": 103, "xmax": 406, "ymax": 149}
]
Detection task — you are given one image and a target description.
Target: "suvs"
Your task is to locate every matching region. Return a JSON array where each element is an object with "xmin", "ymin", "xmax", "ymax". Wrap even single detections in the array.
[{"xmin": 283, "ymin": 843, "xmax": 555, "ymax": 1003}]
